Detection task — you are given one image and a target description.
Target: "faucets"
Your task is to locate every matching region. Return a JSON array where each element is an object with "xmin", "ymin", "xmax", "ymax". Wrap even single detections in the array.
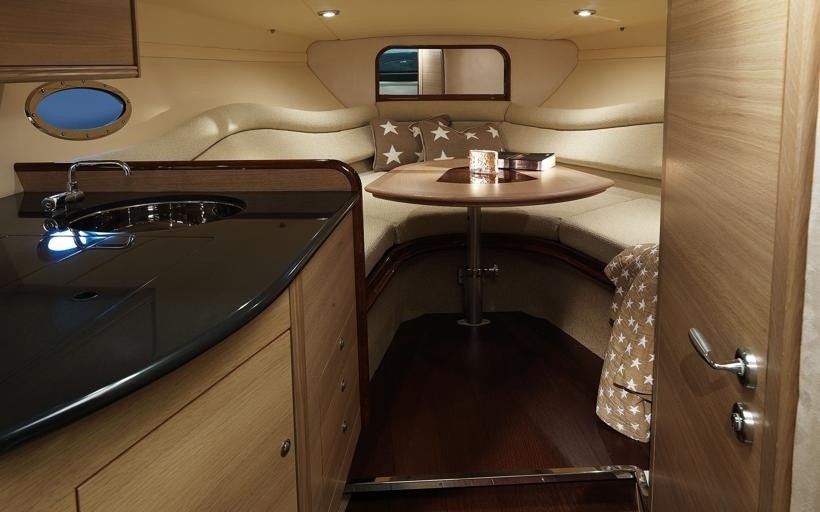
[
  {"xmin": 73, "ymin": 232, "xmax": 136, "ymax": 251},
  {"xmin": 68, "ymin": 158, "xmax": 131, "ymax": 192}
]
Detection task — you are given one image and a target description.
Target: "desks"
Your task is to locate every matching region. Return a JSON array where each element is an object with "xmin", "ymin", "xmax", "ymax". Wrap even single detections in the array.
[{"xmin": 361, "ymin": 156, "xmax": 615, "ymax": 377}]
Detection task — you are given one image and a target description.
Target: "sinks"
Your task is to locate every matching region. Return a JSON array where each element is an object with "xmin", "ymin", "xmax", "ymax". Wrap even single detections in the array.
[{"xmin": 70, "ymin": 197, "xmax": 243, "ymax": 231}]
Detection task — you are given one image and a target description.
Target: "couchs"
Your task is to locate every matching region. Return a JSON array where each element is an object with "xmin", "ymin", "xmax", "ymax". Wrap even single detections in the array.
[{"xmin": 67, "ymin": 97, "xmax": 665, "ymax": 380}]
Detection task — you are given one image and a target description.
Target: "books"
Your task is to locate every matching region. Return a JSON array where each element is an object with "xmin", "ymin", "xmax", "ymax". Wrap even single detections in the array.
[{"xmin": 497, "ymin": 152, "xmax": 557, "ymax": 171}]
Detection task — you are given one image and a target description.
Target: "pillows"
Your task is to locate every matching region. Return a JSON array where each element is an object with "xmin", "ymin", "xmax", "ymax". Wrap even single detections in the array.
[{"xmin": 370, "ymin": 111, "xmax": 507, "ymax": 172}]
